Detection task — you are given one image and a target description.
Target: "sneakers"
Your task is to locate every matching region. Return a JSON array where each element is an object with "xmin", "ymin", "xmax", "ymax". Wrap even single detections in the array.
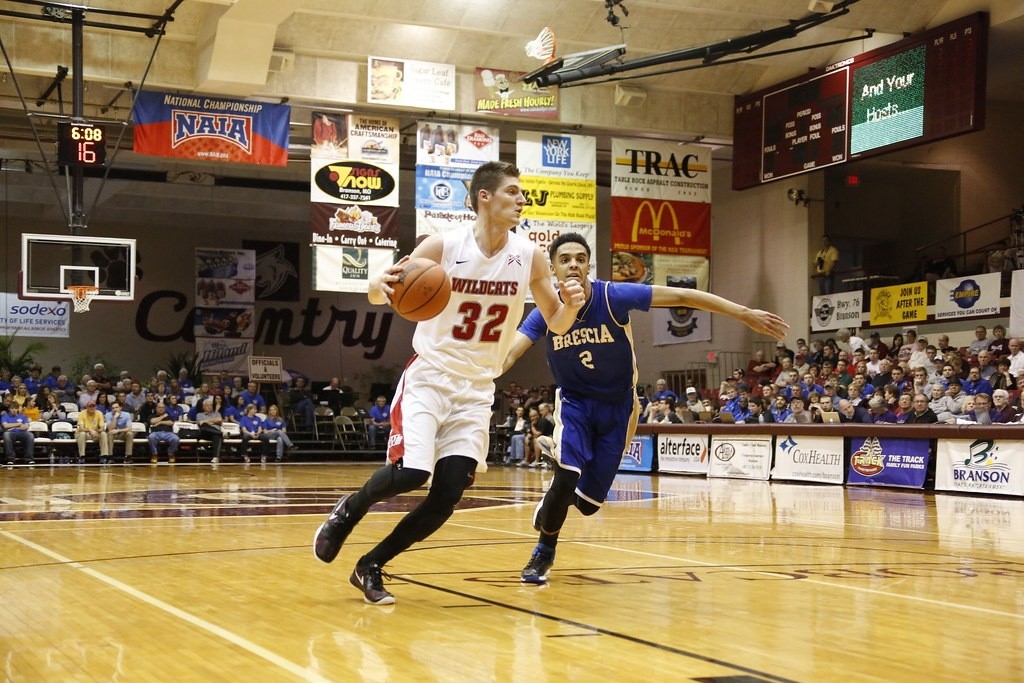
[
  {"xmin": 350, "ymin": 555, "xmax": 396, "ymax": 604},
  {"xmin": 529, "ymin": 460, "xmax": 540, "ymax": 467},
  {"xmin": 520, "ymin": 543, "xmax": 556, "ymax": 581},
  {"xmin": 533, "ymin": 475, "xmax": 555, "ymax": 531},
  {"xmin": 516, "ymin": 460, "xmax": 528, "ymax": 466},
  {"xmin": 314, "ymin": 493, "xmax": 356, "ymax": 563}
]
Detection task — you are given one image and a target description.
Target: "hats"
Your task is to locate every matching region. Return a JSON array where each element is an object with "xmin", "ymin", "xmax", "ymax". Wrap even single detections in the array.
[
  {"xmin": 686, "ymin": 387, "xmax": 696, "ymax": 394},
  {"xmin": 88, "ymin": 401, "xmax": 95, "ymax": 406},
  {"xmin": 825, "ymin": 382, "xmax": 834, "ymax": 388},
  {"xmin": 725, "ymin": 375, "xmax": 736, "ymax": 381},
  {"xmin": 777, "ymin": 342, "xmax": 784, "ymax": 346},
  {"xmin": 918, "ymin": 336, "xmax": 927, "ymax": 342},
  {"xmin": 946, "ymin": 376, "xmax": 963, "ymax": 386},
  {"xmin": 790, "ymin": 396, "xmax": 801, "ymax": 400},
  {"xmin": 870, "ymin": 331, "xmax": 879, "ymax": 337}
]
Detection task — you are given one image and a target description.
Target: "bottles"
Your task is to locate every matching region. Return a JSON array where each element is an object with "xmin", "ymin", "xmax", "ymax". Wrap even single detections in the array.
[
  {"xmin": 758, "ymin": 413, "xmax": 764, "ymax": 422},
  {"xmin": 445, "ymin": 146, "xmax": 450, "ymax": 166},
  {"xmin": 207, "ymin": 279, "xmax": 216, "ymax": 300},
  {"xmin": 428, "ymin": 144, "xmax": 435, "ymax": 163},
  {"xmin": 445, "ymin": 126, "xmax": 459, "ymax": 153},
  {"xmin": 216, "ymin": 292, "xmax": 221, "ymax": 306},
  {"xmin": 420, "ymin": 124, "xmax": 431, "ymax": 149},
  {"xmin": 203, "ymin": 292, "xmax": 208, "ymax": 305},
  {"xmin": 216, "ymin": 281, "xmax": 225, "ymax": 298},
  {"xmin": 431, "ymin": 125, "xmax": 444, "ymax": 156},
  {"xmin": 665, "ymin": 415, "xmax": 668, "ymax": 423},
  {"xmin": 197, "ymin": 279, "xmax": 207, "ymax": 296}
]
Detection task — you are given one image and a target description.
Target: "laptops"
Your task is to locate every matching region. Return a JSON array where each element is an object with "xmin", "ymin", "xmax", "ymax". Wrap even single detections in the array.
[
  {"xmin": 681, "ymin": 410, "xmax": 735, "ymax": 424},
  {"xmin": 822, "ymin": 412, "xmax": 841, "ymax": 423}
]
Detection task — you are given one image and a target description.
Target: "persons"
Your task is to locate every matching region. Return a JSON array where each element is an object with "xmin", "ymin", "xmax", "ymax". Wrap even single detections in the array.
[
  {"xmin": 502, "ymin": 232, "xmax": 790, "ymax": 583},
  {"xmin": 289, "ymin": 378, "xmax": 317, "ymax": 431},
  {"xmin": 494, "ymin": 383, "xmax": 558, "ymax": 469},
  {"xmin": 323, "ymin": 377, "xmax": 343, "ymax": 393},
  {"xmin": 637, "ymin": 327, "xmax": 1024, "ymax": 482},
  {"xmin": 313, "ymin": 160, "xmax": 585, "ymax": 605},
  {"xmin": 368, "ymin": 395, "xmax": 390, "ymax": 450},
  {"xmin": 0, "ymin": 363, "xmax": 298, "ymax": 465}
]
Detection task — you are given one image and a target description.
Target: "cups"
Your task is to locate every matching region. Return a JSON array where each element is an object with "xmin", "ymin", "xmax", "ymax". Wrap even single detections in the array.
[{"xmin": 371, "ymin": 65, "xmax": 402, "ymax": 100}]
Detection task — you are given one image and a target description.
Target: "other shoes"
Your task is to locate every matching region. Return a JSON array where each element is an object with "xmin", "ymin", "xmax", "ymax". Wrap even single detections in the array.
[
  {"xmin": 25, "ymin": 459, "xmax": 35, "ymax": 463},
  {"xmin": 8, "ymin": 459, "xmax": 15, "ymax": 464},
  {"xmin": 371, "ymin": 446, "xmax": 376, "ymax": 450},
  {"xmin": 78, "ymin": 431, "xmax": 299, "ymax": 463}
]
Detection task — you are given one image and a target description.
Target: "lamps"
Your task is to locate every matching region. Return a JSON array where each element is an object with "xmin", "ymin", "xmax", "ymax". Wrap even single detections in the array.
[
  {"xmin": 614, "ymin": 83, "xmax": 647, "ymax": 111},
  {"xmin": 787, "ymin": 187, "xmax": 842, "ymax": 209},
  {"xmin": 268, "ymin": 52, "xmax": 297, "ymax": 73}
]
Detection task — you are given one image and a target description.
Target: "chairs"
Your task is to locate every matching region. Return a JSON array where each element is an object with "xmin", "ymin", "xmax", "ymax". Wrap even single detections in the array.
[
  {"xmin": 700, "ymin": 357, "xmax": 782, "ymax": 401},
  {"xmin": 1007, "ymin": 390, "xmax": 1022, "ymax": 403},
  {"xmin": 5, "ymin": 387, "xmax": 375, "ymax": 457},
  {"xmin": 959, "ymin": 347, "xmax": 1010, "ymax": 370}
]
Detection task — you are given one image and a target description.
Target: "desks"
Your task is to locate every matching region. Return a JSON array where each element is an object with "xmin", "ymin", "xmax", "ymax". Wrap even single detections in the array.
[{"xmin": 842, "ymin": 275, "xmax": 900, "ymax": 313}]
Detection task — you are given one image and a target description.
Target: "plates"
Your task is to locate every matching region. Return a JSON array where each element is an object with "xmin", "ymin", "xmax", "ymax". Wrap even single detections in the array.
[{"xmin": 619, "ymin": 252, "xmax": 647, "ymax": 284}]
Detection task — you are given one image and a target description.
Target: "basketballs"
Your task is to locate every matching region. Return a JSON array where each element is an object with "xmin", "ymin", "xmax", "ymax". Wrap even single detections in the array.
[{"xmin": 388, "ymin": 256, "xmax": 452, "ymax": 323}]
